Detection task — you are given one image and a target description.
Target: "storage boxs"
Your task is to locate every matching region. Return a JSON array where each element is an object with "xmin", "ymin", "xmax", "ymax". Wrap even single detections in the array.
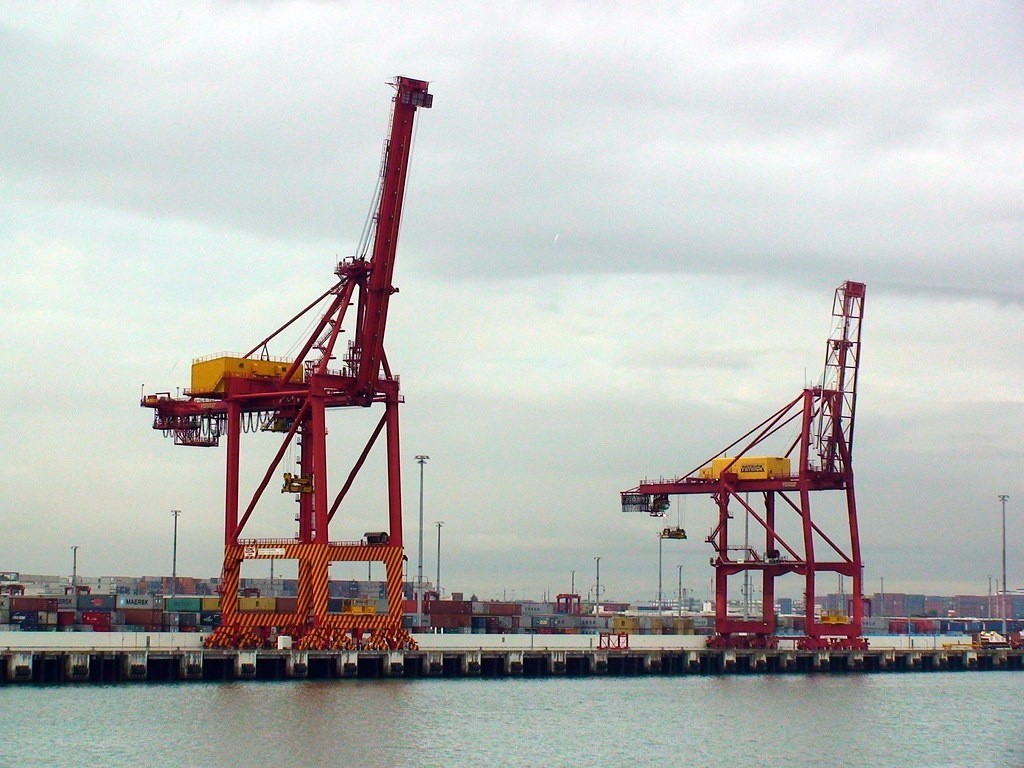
[{"xmin": 0, "ymin": 575, "xmax": 1024, "ymax": 635}]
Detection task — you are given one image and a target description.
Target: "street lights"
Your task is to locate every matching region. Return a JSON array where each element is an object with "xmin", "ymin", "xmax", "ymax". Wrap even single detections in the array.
[
  {"xmin": 592, "ymin": 556, "xmax": 602, "ymax": 617},
  {"xmin": 998, "ymin": 494, "xmax": 1011, "ymax": 643},
  {"xmin": 70, "ymin": 543, "xmax": 83, "ymax": 589},
  {"xmin": 677, "ymin": 565, "xmax": 684, "ymax": 617},
  {"xmin": 414, "ymin": 454, "xmax": 431, "ymax": 628},
  {"xmin": 168, "ymin": 509, "xmax": 183, "ymax": 599},
  {"xmin": 570, "ymin": 569, "xmax": 575, "ymax": 600},
  {"xmin": 432, "ymin": 520, "xmax": 444, "ymax": 600}
]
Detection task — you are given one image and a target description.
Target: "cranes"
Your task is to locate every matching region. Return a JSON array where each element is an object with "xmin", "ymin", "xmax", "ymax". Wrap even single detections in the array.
[
  {"xmin": 622, "ymin": 279, "xmax": 873, "ymax": 653},
  {"xmin": 139, "ymin": 74, "xmax": 436, "ymax": 651}
]
